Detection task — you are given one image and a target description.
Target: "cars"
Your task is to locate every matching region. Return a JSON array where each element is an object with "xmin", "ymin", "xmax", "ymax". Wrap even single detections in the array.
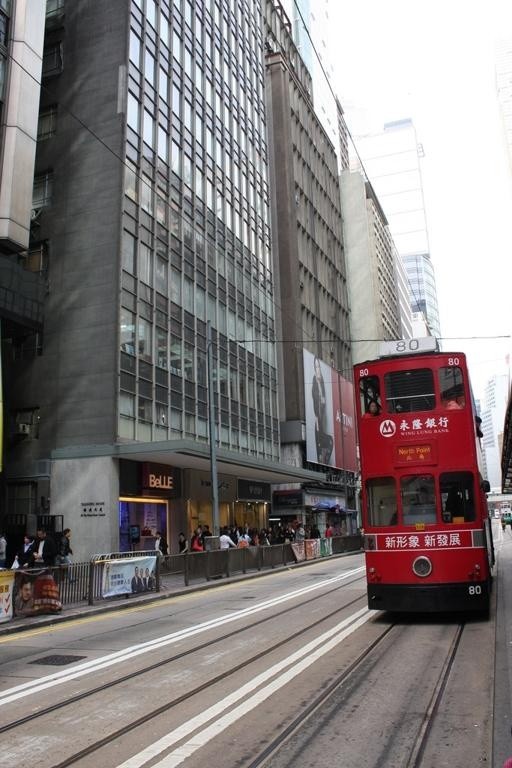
[{"xmin": 500, "ymin": 513, "xmax": 512, "ymax": 531}]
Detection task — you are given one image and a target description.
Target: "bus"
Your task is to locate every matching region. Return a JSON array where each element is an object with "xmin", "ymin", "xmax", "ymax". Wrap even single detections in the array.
[
  {"xmin": 499, "ymin": 501, "xmax": 511, "ymax": 514},
  {"xmin": 352, "ymin": 334, "xmax": 496, "ymax": 625}
]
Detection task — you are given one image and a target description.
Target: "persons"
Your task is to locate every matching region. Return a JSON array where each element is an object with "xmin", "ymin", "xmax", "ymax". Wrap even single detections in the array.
[
  {"xmin": 189, "ymin": 520, "xmax": 323, "ymax": 552},
  {"xmin": 143, "ymin": 567, "xmax": 153, "ymax": 591},
  {"xmin": 138, "ymin": 568, "xmax": 147, "ymax": 591},
  {"xmin": 130, "ymin": 566, "xmax": 143, "ymax": 593},
  {"xmin": 149, "ymin": 570, "xmax": 158, "ymax": 591},
  {"xmin": 474, "ymin": 415, "xmax": 484, "ymax": 438},
  {"xmin": 13, "ymin": 533, "xmax": 38, "ymax": 569},
  {"xmin": 177, "ymin": 532, "xmax": 189, "ymax": 555},
  {"xmin": 0, "ymin": 533, "xmax": 9, "ymax": 568},
  {"xmin": 324, "ymin": 523, "xmax": 334, "ymax": 538},
  {"xmin": 444, "ymin": 392, "xmax": 465, "ymax": 411},
  {"xmin": 311, "ymin": 355, "xmax": 336, "ymax": 464},
  {"xmin": 32, "ymin": 524, "xmax": 57, "ymax": 566},
  {"xmin": 50, "ymin": 527, "xmax": 80, "ymax": 585},
  {"xmin": 154, "ymin": 532, "xmax": 172, "ymax": 571},
  {"xmin": 405, "ymin": 485, "xmax": 437, "ymax": 519},
  {"xmin": 13, "ymin": 575, "xmax": 34, "ymax": 611},
  {"xmin": 361, "ymin": 400, "xmax": 380, "ymax": 419}
]
